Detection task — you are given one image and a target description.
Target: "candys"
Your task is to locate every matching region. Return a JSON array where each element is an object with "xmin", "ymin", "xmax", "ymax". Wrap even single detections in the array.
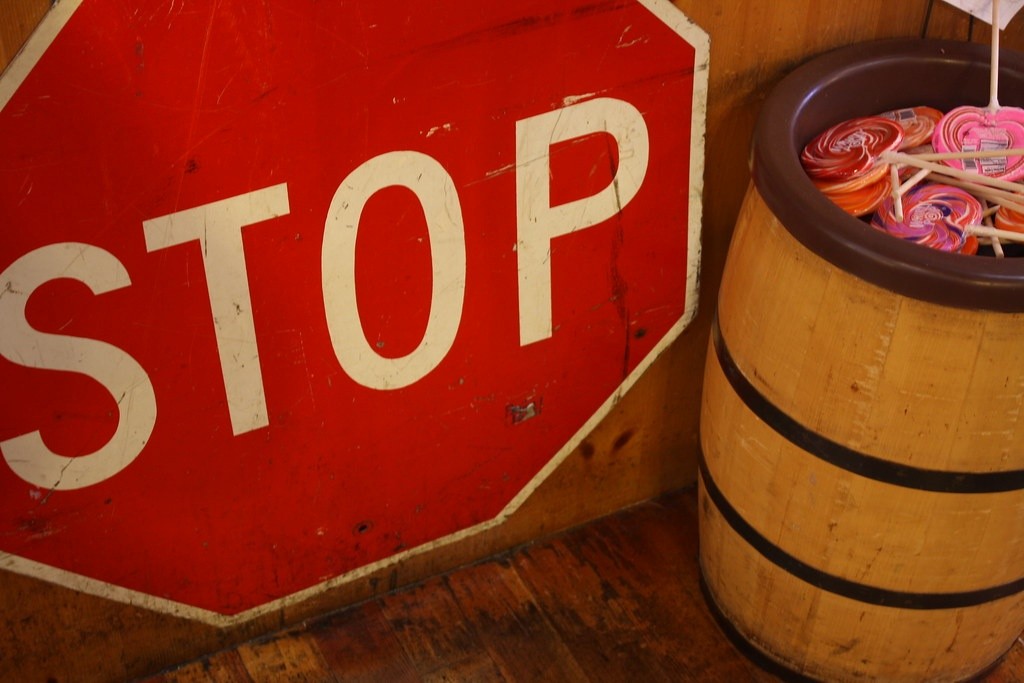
[{"xmin": 798, "ymin": 106, "xmax": 1024, "ymax": 257}]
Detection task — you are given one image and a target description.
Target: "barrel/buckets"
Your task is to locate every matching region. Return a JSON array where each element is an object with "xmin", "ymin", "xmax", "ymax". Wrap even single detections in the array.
[{"xmin": 696, "ymin": 34, "xmax": 1024, "ymax": 683}]
